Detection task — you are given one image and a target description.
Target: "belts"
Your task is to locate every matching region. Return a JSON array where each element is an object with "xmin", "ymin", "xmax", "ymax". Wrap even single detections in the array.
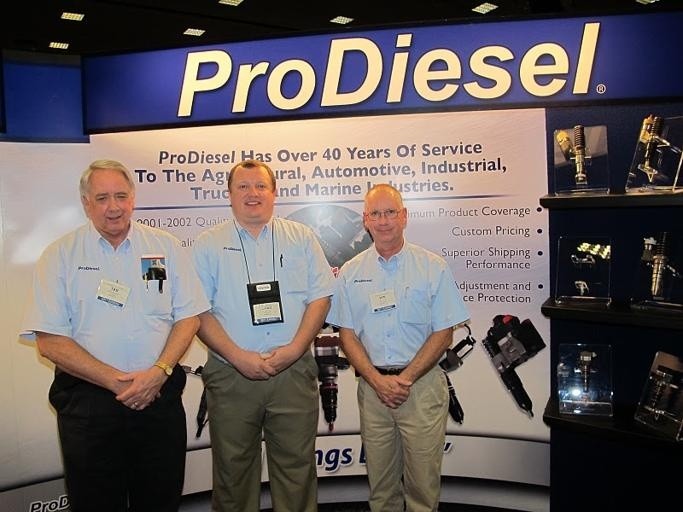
[{"xmin": 375, "ymin": 368, "xmax": 403, "ymax": 375}]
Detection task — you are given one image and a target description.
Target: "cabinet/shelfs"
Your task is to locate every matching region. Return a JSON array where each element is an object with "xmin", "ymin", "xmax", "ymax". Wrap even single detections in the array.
[{"xmin": 537, "ymin": 191, "xmax": 683, "ymax": 446}]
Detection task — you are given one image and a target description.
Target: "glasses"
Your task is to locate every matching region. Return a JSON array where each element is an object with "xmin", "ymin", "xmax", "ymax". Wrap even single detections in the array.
[{"xmin": 365, "ymin": 209, "xmax": 399, "ymax": 219}]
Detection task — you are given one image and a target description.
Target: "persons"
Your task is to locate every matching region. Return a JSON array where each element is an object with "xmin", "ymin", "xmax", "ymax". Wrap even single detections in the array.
[
  {"xmin": 189, "ymin": 160, "xmax": 337, "ymax": 511},
  {"xmin": 18, "ymin": 158, "xmax": 212, "ymax": 511},
  {"xmin": 325, "ymin": 184, "xmax": 470, "ymax": 512}
]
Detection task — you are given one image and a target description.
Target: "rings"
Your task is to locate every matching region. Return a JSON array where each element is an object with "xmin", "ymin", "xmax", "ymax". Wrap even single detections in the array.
[{"xmin": 133, "ymin": 401, "xmax": 138, "ymax": 408}]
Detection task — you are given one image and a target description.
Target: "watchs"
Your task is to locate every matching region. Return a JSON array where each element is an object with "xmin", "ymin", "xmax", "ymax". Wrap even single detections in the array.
[{"xmin": 153, "ymin": 361, "xmax": 173, "ymax": 376}]
